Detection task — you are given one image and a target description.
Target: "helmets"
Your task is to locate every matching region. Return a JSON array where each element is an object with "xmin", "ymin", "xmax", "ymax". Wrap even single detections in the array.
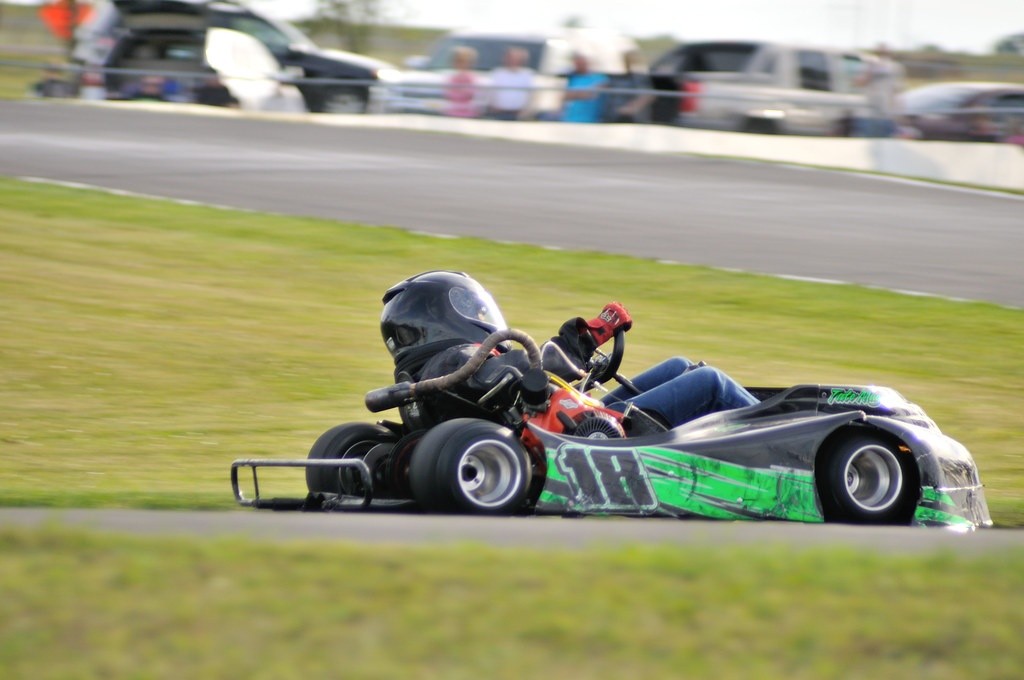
[{"xmin": 380, "ymin": 270, "xmax": 512, "ymax": 361}]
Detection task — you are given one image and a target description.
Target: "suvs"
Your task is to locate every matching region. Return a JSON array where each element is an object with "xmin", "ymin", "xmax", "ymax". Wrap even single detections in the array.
[
  {"xmin": 384, "ymin": 25, "xmax": 635, "ymax": 119},
  {"xmin": 102, "ymin": 0, "xmax": 385, "ymax": 116}
]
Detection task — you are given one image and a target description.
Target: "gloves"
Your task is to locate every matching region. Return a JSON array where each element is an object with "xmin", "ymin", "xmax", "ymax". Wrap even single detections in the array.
[{"xmin": 581, "ymin": 301, "xmax": 632, "ymax": 346}]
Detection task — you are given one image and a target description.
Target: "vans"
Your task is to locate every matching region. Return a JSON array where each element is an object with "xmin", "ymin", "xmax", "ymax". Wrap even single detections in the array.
[{"xmin": 649, "ymin": 42, "xmax": 883, "ymax": 136}]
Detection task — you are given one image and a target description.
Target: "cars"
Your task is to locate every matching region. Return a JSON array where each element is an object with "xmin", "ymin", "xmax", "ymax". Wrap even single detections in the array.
[{"xmin": 893, "ymin": 81, "xmax": 1024, "ymax": 145}]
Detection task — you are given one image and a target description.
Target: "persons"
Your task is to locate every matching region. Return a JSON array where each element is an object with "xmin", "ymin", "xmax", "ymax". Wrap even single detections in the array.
[
  {"xmin": 853, "ymin": 42, "xmax": 1024, "ymax": 143},
  {"xmin": 440, "ymin": 44, "xmax": 656, "ymax": 124},
  {"xmin": 36, "ymin": 69, "xmax": 193, "ymax": 102},
  {"xmin": 380, "ymin": 269, "xmax": 763, "ymax": 441}
]
